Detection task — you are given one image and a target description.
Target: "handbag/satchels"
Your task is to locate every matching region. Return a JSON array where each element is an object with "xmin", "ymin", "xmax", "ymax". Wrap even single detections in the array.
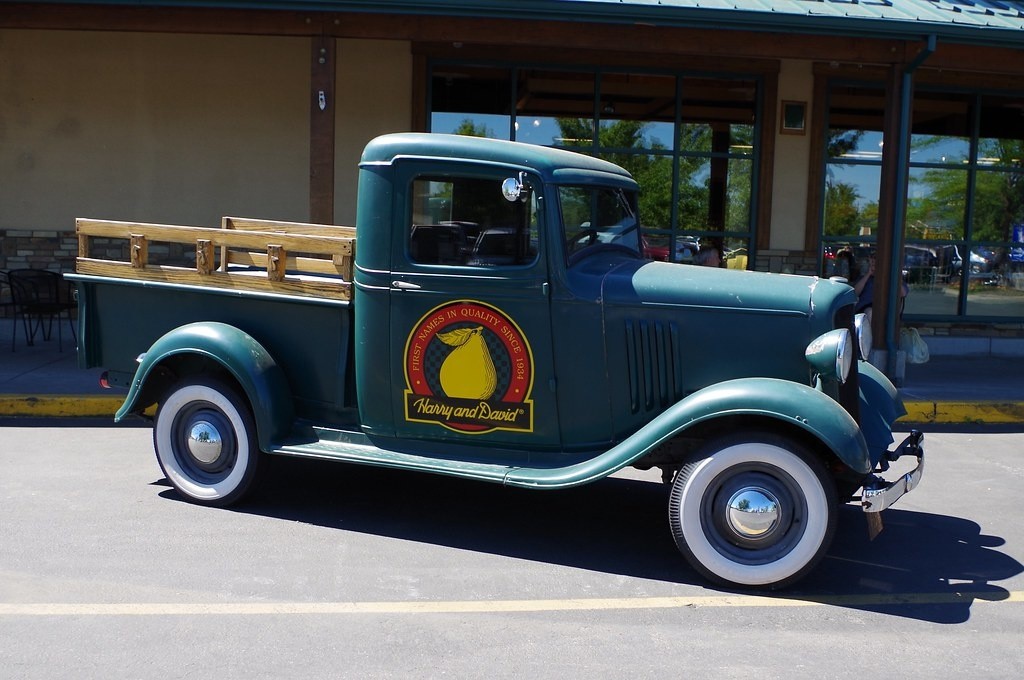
[{"xmin": 900, "ymin": 327, "xmax": 929, "ymax": 363}]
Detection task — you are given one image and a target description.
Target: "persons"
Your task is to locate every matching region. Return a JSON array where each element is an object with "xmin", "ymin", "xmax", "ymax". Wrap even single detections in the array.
[
  {"xmin": 851, "ymin": 249, "xmax": 908, "ymax": 316},
  {"xmin": 692, "ymin": 236, "xmax": 721, "ymax": 267}
]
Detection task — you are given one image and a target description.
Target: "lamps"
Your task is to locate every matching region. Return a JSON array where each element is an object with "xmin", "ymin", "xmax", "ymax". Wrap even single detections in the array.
[
  {"xmin": 515, "ymin": 69, "xmax": 548, "ymax": 113},
  {"xmin": 601, "ymin": 98, "xmax": 616, "ymax": 115}
]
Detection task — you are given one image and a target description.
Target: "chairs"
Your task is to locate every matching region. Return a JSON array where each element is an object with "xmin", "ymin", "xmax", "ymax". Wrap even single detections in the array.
[
  {"xmin": 0, "ymin": 268, "xmax": 80, "ymax": 353},
  {"xmin": 410, "ymin": 221, "xmax": 519, "ymax": 266}
]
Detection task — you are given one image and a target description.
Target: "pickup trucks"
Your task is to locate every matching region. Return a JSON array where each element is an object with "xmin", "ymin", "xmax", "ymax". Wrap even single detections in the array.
[{"xmin": 62, "ymin": 132, "xmax": 925, "ymax": 595}]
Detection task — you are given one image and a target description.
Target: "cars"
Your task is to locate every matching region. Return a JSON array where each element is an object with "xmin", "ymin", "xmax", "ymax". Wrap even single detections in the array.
[
  {"xmin": 822, "ymin": 239, "xmax": 991, "ymax": 288},
  {"xmin": 638, "ymin": 234, "xmax": 707, "ymax": 263}
]
